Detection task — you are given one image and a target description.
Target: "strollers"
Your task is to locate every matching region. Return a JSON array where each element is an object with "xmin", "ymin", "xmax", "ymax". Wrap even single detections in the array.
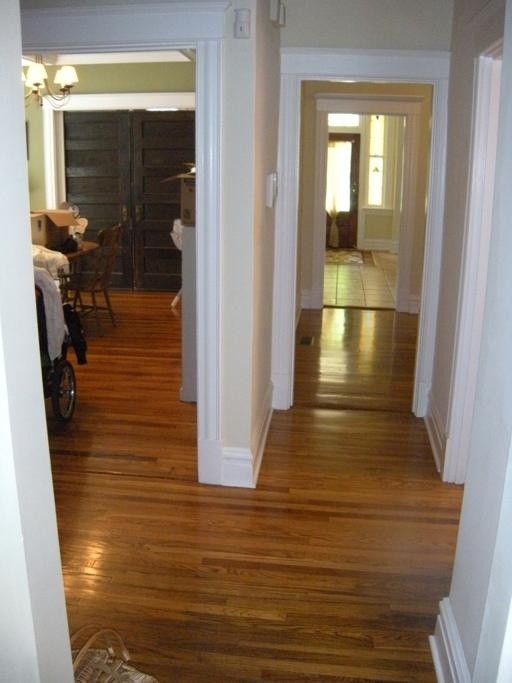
[{"xmin": 32, "ymin": 265, "xmax": 88, "ymax": 423}]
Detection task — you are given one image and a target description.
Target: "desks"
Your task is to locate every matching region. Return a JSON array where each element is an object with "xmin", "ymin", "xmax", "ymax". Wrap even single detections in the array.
[{"xmin": 31, "ymin": 237, "xmax": 99, "ymax": 299}]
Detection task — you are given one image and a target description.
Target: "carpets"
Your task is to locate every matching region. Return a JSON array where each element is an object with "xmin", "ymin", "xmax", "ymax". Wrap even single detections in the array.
[{"xmin": 325, "ymin": 249, "xmax": 375, "ymax": 267}]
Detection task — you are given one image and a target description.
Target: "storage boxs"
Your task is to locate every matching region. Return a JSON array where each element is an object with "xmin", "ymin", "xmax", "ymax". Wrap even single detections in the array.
[
  {"xmin": 178, "ymin": 163, "xmax": 195, "ymax": 226},
  {"xmin": 31, "ymin": 208, "xmax": 80, "ymax": 250}
]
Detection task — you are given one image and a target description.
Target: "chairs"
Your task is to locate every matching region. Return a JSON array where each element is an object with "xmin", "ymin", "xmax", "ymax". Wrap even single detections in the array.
[{"xmin": 64, "ymin": 222, "xmax": 123, "ymax": 336}]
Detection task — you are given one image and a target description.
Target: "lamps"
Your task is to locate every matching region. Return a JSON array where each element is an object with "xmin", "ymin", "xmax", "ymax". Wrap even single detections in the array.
[{"xmin": 21, "ymin": 55, "xmax": 80, "ymax": 109}]
